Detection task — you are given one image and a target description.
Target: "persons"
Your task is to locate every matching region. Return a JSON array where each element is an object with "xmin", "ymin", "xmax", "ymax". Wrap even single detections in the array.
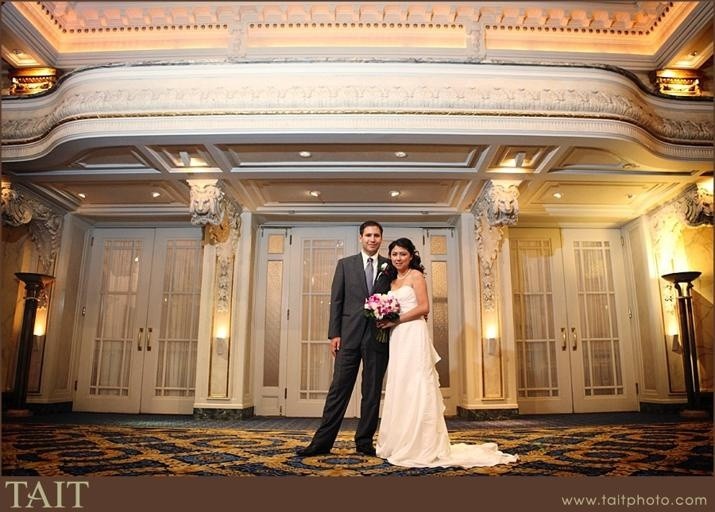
[
  {"xmin": 295, "ymin": 220, "xmax": 397, "ymax": 457},
  {"xmin": 376, "ymin": 238, "xmax": 450, "ymax": 469}
]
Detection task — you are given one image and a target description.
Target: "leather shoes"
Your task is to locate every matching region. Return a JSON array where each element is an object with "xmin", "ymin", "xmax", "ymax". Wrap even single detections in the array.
[
  {"xmin": 356, "ymin": 446, "xmax": 376, "ymax": 456},
  {"xmin": 296, "ymin": 444, "xmax": 330, "ymax": 456}
]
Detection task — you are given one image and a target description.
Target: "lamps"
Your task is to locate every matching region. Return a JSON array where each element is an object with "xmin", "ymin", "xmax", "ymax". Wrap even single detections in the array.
[{"xmin": 23, "ymin": 292, "xmax": 50, "ymax": 336}]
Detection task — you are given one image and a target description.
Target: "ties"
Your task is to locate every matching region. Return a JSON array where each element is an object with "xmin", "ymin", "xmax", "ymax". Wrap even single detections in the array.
[{"xmin": 366, "ymin": 258, "xmax": 373, "ymax": 295}]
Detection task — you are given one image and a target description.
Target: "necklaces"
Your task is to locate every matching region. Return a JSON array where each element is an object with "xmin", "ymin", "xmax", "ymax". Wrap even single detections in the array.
[{"xmin": 395, "ymin": 268, "xmax": 412, "ymax": 280}]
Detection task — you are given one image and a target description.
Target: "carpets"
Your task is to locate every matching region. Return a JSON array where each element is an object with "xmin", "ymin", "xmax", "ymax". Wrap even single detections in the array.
[{"xmin": 0, "ymin": 424, "xmax": 713, "ymax": 479}]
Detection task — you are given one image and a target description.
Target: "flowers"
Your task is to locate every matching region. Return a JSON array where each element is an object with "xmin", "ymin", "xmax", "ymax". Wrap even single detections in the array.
[{"xmin": 362, "ymin": 291, "xmax": 401, "ymax": 344}]
